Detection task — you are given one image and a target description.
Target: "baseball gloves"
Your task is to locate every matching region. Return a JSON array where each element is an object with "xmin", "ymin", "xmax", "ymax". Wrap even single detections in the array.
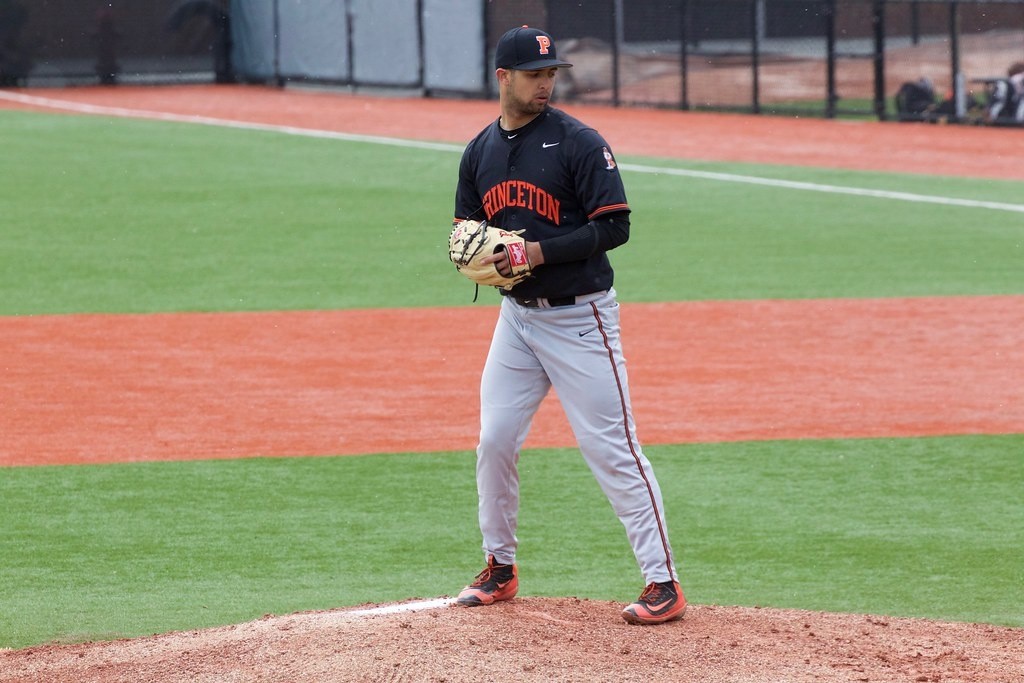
[{"xmin": 449, "ymin": 220, "xmax": 534, "ymax": 291}]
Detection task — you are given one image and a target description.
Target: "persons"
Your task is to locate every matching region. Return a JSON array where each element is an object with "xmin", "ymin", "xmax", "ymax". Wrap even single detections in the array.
[
  {"xmin": 448, "ymin": 26, "xmax": 688, "ymax": 624},
  {"xmin": 897, "ymin": 61, "xmax": 1023, "ymax": 125}
]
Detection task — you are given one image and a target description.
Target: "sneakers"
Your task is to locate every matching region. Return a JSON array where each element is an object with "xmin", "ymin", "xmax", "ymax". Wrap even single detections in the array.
[
  {"xmin": 456, "ymin": 554, "xmax": 520, "ymax": 606},
  {"xmin": 621, "ymin": 581, "xmax": 688, "ymax": 624}
]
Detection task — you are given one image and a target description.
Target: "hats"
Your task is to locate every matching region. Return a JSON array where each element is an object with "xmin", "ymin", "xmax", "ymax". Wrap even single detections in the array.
[{"xmin": 495, "ymin": 25, "xmax": 573, "ymax": 70}]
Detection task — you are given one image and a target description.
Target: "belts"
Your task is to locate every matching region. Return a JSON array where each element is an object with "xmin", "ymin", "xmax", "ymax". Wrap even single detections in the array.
[{"xmin": 510, "ymin": 287, "xmax": 611, "ymax": 308}]
